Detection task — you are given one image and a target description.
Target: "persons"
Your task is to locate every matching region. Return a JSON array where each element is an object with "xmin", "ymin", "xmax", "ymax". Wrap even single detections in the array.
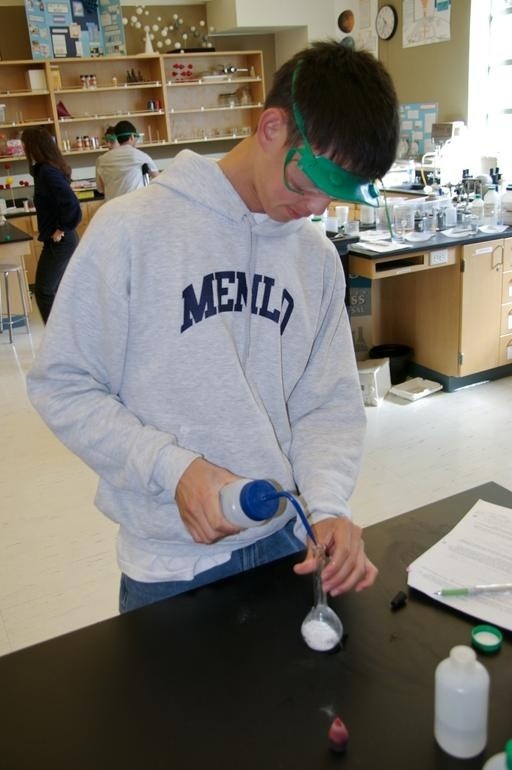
[
  {"xmin": 103, "ymin": 125, "xmax": 121, "ymax": 151},
  {"xmin": 19, "ymin": 125, "xmax": 83, "ymax": 326},
  {"xmin": 22, "ymin": 36, "xmax": 404, "ymax": 618},
  {"xmin": 93, "ymin": 120, "xmax": 161, "ymax": 203}
]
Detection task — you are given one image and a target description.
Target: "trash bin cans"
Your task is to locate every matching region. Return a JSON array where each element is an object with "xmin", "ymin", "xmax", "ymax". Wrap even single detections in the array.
[{"xmin": 368, "ymin": 343, "xmax": 414, "ymax": 385}]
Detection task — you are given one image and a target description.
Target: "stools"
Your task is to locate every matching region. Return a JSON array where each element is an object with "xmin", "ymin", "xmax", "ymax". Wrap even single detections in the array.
[{"xmin": 1, "ymin": 263, "xmax": 31, "ymax": 344}]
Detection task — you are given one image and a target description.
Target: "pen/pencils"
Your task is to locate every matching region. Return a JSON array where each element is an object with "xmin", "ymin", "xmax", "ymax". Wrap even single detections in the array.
[{"xmin": 434, "ymin": 583, "xmax": 512, "ymax": 597}]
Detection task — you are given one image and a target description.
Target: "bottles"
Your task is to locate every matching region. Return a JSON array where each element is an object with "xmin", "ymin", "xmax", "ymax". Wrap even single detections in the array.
[
  {"xmin": 111, "ymin": 75, "xmax": 118, "ymax": 87},
  {"xmin": 329, "ymin": 717, "xmax": 350, "ymax": 755},
  {"xmin": 218, "ymin": 87, "xmax": 250, "ymax": 107},
  {"xmin": 300, "ymin": 557, "xmax": 344, "ymax": 651},
  {"xmin": 79, "ymin": 73, "xmax": 97, "ymax": 88},
  {"xmin": 398, "ymin": 133, "xmax": 409, "ymax": 159},
  {"xmin": 62, "ymin": 135, "xmax": 99, "ymax": 151},
  {"xmin": 147, "ymin": 98, "xmax": 160, "ymax": 112},
  {"xmin": 250, "ymin": 66, "xmax": 256, "ymax": 79},
  {"xmin": 360, "ymin": 181, "xmax": 378, "ymax": 206},
  {"xmin": 0, "ymin": 104, "xmax": 23, "ymax": 124},
  {"xmin": 221, "ymin": 479, "xmax": 316, "ymax": 553},
  {"xmin": 471, "ymin": 185, "xmax": 512, "ymax": 229},
  {"xmin": 1, "ymin": 132, "xmax": 7, "ymax": 156},
  {"xmin": 434, "ymin": 645, "xmax": 512, "ymax": 770}
]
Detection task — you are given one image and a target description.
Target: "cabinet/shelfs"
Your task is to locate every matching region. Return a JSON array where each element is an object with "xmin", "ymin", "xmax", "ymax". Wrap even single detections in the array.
[
  {"xmin": 349, "ymin": 236, "xmax": 512, "ymax": 392},
  {"xmin": 0, "ymin": 50, "xmax": 266, "ymax": 162}
]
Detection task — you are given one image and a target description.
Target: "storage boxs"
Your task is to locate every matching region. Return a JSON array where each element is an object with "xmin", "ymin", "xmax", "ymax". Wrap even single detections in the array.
[{"xmin": 354, "ymin": 358, "xmax": 392, "ymax": 407}]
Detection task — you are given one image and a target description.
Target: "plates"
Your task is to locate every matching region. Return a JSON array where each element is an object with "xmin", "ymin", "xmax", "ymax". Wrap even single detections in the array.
[{"xmin": 199, "ymin": 75, "xmax": 228, "ymax": 82}]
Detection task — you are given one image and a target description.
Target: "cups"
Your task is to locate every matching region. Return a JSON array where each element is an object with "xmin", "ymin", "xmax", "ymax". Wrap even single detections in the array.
[
  {"xmin": 199, "ymin": 127, "xmax": 252, "ymax": 137},
  {"xmin": 325, "ymin": 198, "xmax": 478, "ymax": 242}
]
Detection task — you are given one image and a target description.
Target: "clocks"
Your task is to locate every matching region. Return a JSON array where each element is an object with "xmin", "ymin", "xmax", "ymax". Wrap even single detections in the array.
[{"xmin": 374, "ymin": 5, "xmax": 397, "ymax": 40}]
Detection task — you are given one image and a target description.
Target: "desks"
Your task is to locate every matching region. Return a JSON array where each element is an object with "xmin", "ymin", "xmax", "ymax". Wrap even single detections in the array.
[{"xmin": 0, "ymin": 481, "xmax": 511, "ymax": 768}]
